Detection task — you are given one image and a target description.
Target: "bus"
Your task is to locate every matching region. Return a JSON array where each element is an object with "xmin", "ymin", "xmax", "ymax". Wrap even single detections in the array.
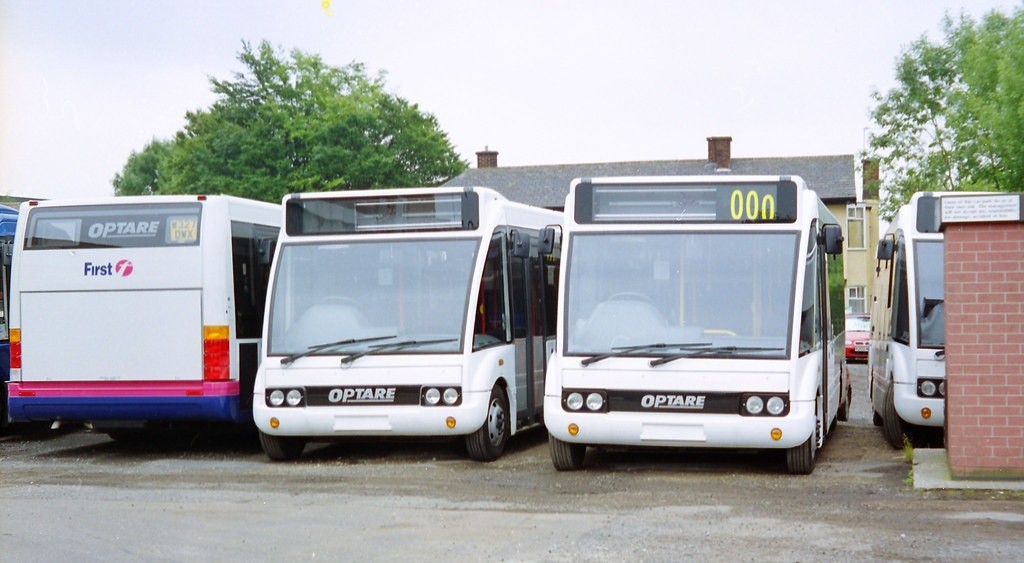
[
  {"xmin": 537, "ymin": 174, "xmax": 848, "ymax": 476},
  {"xmin": 865, "ymin": 189, "xmax": 1002, "ymax": 453},
  {"xmin": 251, "ymin": 185, "xmax": 564, "ymax": 462},
  {"xmin": 0, "ymin": 204, "xmax": 76, "ymax": 435},
  {"xmin": 6, "ymin": 191, "xmax": 285, "ymax": 447}
]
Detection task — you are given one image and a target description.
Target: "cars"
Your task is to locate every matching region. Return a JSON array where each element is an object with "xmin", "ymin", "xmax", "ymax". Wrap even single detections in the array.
[{"xmin": 844, "ymin": 311, "xmax": 873, "ymax": 362}]
